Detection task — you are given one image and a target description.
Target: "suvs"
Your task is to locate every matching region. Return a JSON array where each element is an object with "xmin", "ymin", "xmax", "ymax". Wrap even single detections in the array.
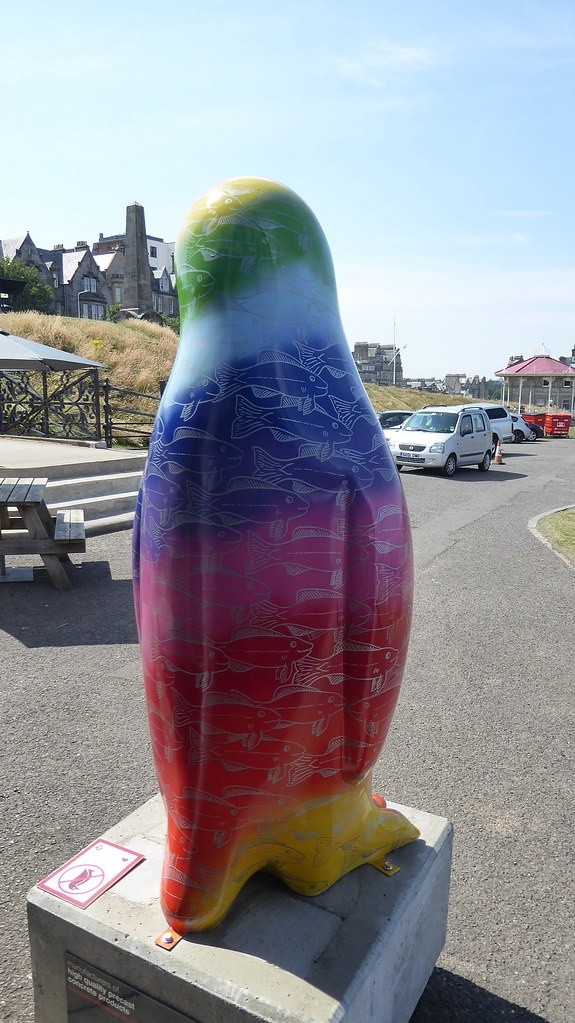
[{"xmin": 389, "ymin": 404, "xmax": 492, "ymax": 476}]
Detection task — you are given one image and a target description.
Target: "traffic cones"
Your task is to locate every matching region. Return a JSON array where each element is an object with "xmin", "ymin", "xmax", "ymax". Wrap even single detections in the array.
[{"xmin": 493, "ymin": 441, "xmax": 506, "ymax": 465}]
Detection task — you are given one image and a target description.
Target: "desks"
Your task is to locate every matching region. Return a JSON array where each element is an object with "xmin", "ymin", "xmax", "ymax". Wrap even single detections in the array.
[{"xmin": 0, "ymin": 478, "xmax": 78, "ymax": 591}]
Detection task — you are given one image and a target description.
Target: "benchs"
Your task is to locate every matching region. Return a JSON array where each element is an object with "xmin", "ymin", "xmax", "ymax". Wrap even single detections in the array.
[{"xmin": 54, "ymin": 510, "xmax": 88, "ymax": 554}]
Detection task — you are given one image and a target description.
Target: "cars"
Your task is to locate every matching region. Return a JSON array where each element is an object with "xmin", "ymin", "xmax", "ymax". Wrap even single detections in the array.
[
  {"xmin": 510, "ymin": 413, "xmax": 531, "ymax": 443},
  {"xmin": 378, "ymin": 410, "xmax": 414, "ymax": 443},
  {"xmin": 528, "ymin": 424, "xmax": 543, "ymax": 441},
  {"xmin": 426, "ymin": 403, "xmax": 515, "ymax": 456}
]
